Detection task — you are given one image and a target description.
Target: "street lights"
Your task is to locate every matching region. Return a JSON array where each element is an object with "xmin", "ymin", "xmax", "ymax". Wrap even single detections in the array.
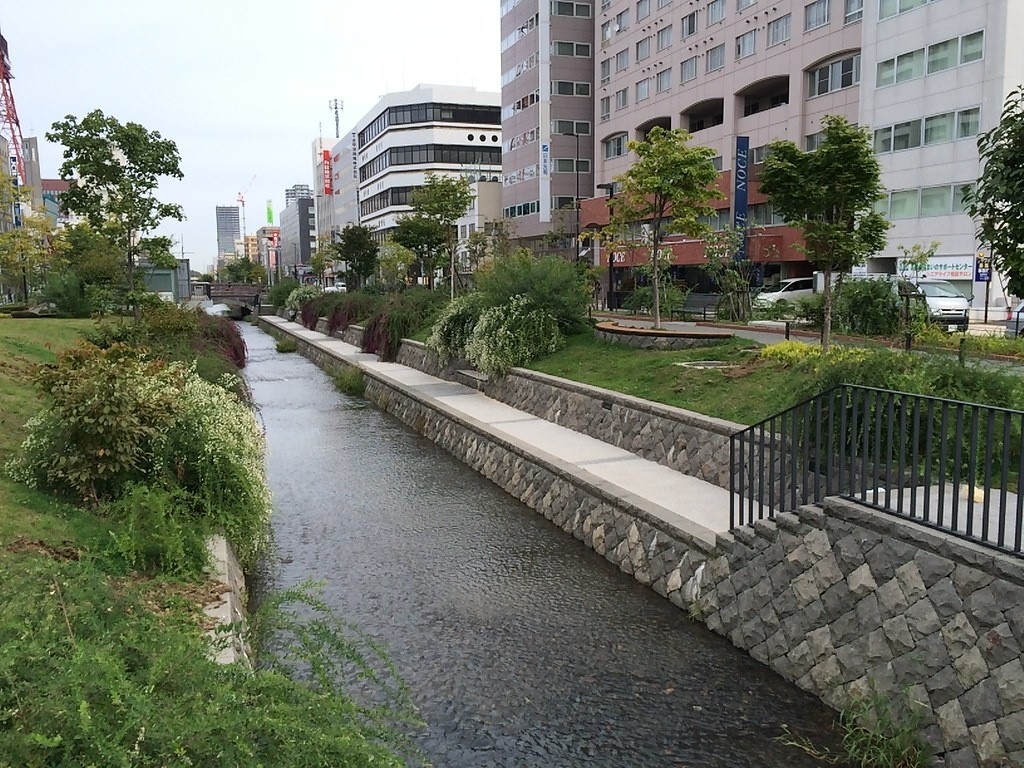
[
  {"xmin": 594, "ymin": 183, "xmax": 615, "ymax": 311},
  {"xmin": 563, "ymin": 134, "xmax": 581, "ymax": 265}
]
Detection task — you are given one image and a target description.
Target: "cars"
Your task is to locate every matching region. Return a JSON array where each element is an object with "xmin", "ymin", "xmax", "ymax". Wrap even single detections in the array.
[
  {"xmin": 1006, "ymin": 306, "xmax": 1024, "ymax": 339},
  {"xmin": 303, "ymin": 276, "xmax": 346, "ymax": 296}
]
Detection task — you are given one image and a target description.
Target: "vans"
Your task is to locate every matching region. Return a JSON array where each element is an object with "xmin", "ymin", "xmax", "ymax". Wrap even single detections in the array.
[
  {"xmin": 890, "ymin": 276, "xmax": 975, "ymax": 338},
  {"xmin": 752, "ymin": 277, "xmax": 825, "ymax": 313}
]
